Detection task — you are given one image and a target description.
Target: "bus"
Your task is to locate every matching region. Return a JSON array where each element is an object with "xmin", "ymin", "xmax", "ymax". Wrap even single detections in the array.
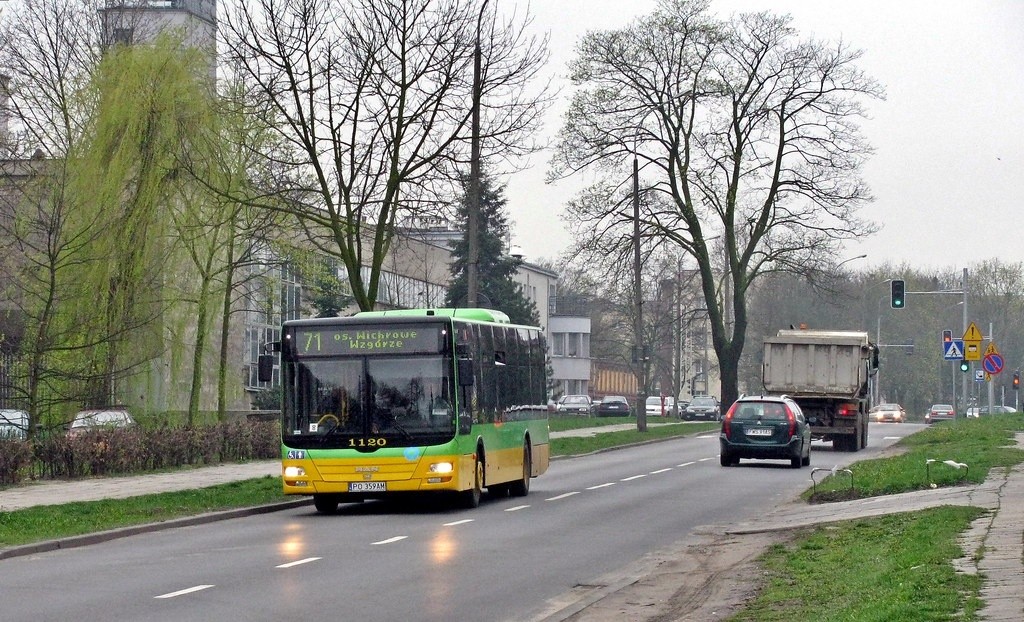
[{"xmin": 257, "ymin": 308, "xmax": 550, "ymax": 515}]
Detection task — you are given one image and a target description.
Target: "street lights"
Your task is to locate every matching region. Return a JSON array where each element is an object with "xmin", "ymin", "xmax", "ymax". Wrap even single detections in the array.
[{"xmin": 633, "ymin": 88, "xmax": 692, "ymax": 431}]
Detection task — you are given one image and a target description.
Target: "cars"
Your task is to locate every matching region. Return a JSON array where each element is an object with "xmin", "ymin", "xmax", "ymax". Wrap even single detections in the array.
[
  {"xmin": 878, "ymin": 403, "xmax": 906, "ymax": 424},
  {"xmin": 924, "ymin": 407, "xmax": 931, "ymax": 424},
  {"xmin": 929, "ymin": 404, "xmax": 955, "ymax": 423},
  {"xmin": 555, "ymin": 393, "xmax": 722, "ymax": 419},
  {"xmin": 0, "ymin": 407, "xmax": 43, "ymax": 443},
  {"xmin": 966, "ymin": 407, "xmax": 981, "ymax": 418},
  {"xmin": 62, "ymin": 409, "xmax": 139, "ymax": 440},
  {"xmin": 868, "ymin": 405, "xmax": 879, "ymax": 422}
]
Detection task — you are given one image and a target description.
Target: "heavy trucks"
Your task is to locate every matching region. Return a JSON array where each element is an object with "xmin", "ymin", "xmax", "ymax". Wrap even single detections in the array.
[{"xmin": 761, "ymin": 324, "xmax": 879, "ymax": 452}]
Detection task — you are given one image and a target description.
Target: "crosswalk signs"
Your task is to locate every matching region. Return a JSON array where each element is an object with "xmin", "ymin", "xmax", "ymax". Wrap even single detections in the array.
[{"xmin": 945, "ymin": 341, "xmax": 963, "ymax": 360}]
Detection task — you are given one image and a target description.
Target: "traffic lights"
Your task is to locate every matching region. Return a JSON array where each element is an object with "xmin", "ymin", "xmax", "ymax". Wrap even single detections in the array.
[
  {"xmin": 959, "ymin": 359, "xmax": 969, "ymax": 372},
  {"xmin": 889, "ymin": 279, "xmax": 906, "ymax": 310},
  {"xmin": 1013, "ymin": 373, "xmax": 1020, "ymax": 391},
  {"xmin": 941, "ymin": 330, "xmax": 952, "ymax": 350}
]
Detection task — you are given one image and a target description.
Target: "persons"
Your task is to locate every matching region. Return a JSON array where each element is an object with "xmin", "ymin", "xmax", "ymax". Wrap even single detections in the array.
[{"xmin": 411, "ymin": 382, "xmax": 452, "ymax": 416}]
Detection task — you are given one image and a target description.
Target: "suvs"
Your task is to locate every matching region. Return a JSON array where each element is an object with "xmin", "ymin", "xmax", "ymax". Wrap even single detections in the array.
[
  {"xmin": 718, "ymin": 393, "xmax": 811, "ymax": 468},
  {"xmin": 978, "ymin": 406, "xmax": 1016, "ymax": 416}
]
